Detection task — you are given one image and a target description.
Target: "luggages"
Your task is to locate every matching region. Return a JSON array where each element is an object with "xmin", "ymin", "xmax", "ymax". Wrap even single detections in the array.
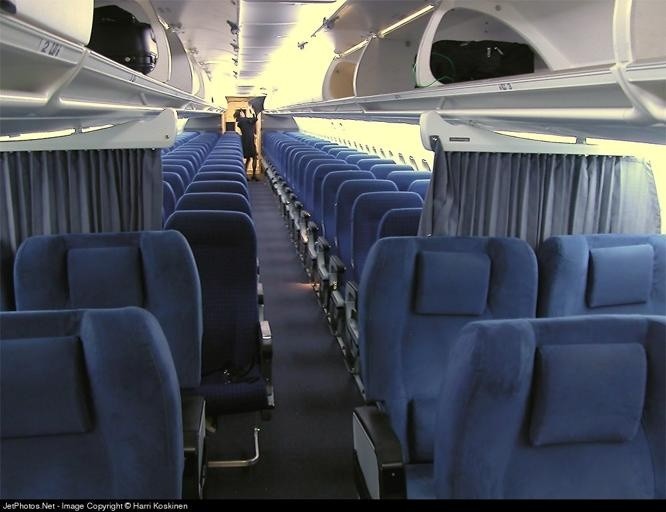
[
  {"xmin": 413, "ymin": 40, "xmax": 534, "ymax": 88},
  {"xmin": 90, "ymin": 4, "xmax": 158, "ymax": 75},
  {"xmin": 248, "ymin": 95, "xmax": 267, "ymax": 114}
]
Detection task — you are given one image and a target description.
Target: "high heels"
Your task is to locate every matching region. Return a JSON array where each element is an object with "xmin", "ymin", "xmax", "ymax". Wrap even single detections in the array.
[{"xmin": 247, "ymin": 175, "xmax": 260, "ymax": 182}]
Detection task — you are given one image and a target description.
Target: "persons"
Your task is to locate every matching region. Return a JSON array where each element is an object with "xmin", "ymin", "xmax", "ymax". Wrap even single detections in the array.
[{"xmin": 232, "ymin": 106, "xmax": 259, "ymax": 183}]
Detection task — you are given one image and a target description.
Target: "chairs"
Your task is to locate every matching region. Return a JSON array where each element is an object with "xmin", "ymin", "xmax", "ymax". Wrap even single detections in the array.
[
  {"xmin": 0, "ymin": 130, "xmax": 273, "ymax": 500},
  {"xmin": 260, "ymin": 128, "xmax": 665, "ymax": 500}
]
